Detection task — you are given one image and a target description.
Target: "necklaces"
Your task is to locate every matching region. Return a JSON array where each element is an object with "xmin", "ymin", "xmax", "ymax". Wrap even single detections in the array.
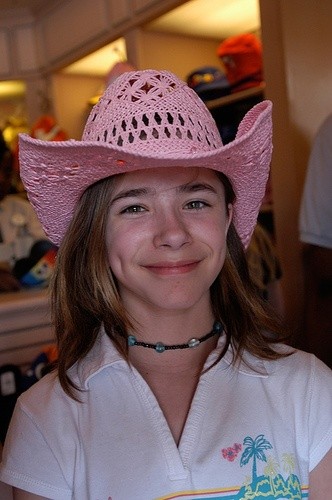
[{"xmin": 125, "ymin": 320, "xmax": 222, "ymax": 354}]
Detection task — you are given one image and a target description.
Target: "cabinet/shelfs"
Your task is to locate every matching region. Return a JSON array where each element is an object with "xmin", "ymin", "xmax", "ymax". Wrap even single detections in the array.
[
  {"xmin": 40, "ymin": 0, "xmax": 293, "ymax": 347},
  {"xmin": 0, "ymin": 0, "xmax": 161, "ymax": 85}
]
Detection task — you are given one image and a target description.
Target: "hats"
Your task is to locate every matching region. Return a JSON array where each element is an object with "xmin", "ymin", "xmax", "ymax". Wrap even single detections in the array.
[
  {"xmin": 187, "ymin": 66, "xmax": 227, "ymax": 102},
  {"xmin": 106, "ymin": 62, "xmax": 139, "ymax": 91},
  {"xmin": 18, "ymin": 69, "xmax": 274, "ymax": 254},
  {"xmin": 216, "ymin": 34, "xmax": 262, "ymax": 92}
]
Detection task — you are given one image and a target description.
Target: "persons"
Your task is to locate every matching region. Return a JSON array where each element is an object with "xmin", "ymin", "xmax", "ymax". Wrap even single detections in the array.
[{"xmin": 0, "ymin": 70, "xmax": 332, "ymax": 500}]
